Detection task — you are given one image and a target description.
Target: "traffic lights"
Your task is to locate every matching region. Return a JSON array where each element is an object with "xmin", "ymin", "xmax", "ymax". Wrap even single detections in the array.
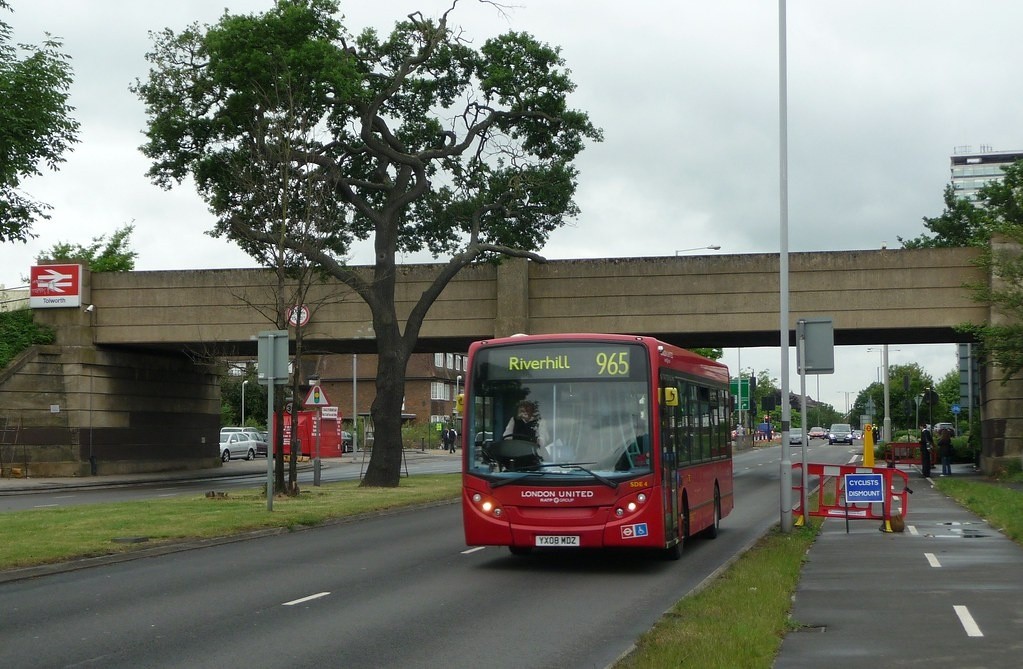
[{"xmin": 764, "ymin": 415, "xmax": 768, "ymax": 423}]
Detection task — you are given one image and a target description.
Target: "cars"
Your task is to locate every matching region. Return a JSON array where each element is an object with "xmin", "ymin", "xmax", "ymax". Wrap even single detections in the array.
[
  {"xmin": 808, "ymin": 427, "xmax": 826, "ymax": 440},
  {"xmin": 789, "ymin": 428, "xmax": 810, "ymax": 446},
  {"xmin": 757, "ymin": 422, "xmax": 774, "ymax": 435},
  {"xmin": 221, "ymin": 426, "xmax": 268, "ymax": 458},
  {"xmin": 341, "ymin": 430, "xmax": 353, "ymax": 452},
  {"xmin": 220, "ymin": 432, "xmax": 258, "ymax": 462}
]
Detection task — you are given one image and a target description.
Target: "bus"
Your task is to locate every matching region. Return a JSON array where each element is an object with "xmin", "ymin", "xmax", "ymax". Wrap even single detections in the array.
[
  {"xmin": 934, "ymin": 423, "xmax": 954, "ymax": 435},
  {"xmin": 455, "ymin": 333, "xmax": 735, "ymax": 559}
]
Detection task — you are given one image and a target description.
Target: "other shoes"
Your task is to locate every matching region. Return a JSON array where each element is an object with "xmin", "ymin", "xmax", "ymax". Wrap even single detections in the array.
[
  {"xmin": 920, "ymin": 474, "xmax": 930, "ymax": 478},
  {"xmin": 939, "ymin": 472, "xmax": 949, "ymax": 477}
]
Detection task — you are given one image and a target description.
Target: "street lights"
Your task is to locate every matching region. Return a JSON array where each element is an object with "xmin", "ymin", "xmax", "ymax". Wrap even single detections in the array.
[
  {"xmin": 456, "ymin": 375, "xmax": 462, "ymax": 418},
  {"xmin": 926, "ymin": 387, "xmax": 932, "ymax": 426},
  {"xmin": 747, "ymin": 366, "xmax": 754, "ymax": 377},
  {"xmin": 867, "ymin": 347, "xmax": 883, "ymax": 383},
  {"xmin": 675, "ymin": 245, "xmax": 721, "ymax": 256},
  {"xmin": 242, "ymin": 379, "xmax": 249, "ymax": 427},
  {"xmin": 837, "ymin": 391, "xmax": 855, "ymax": 412}
]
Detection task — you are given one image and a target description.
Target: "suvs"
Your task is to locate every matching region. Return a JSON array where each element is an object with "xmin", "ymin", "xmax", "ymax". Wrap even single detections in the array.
[{"xmin": 826, "ymin": 424, "xmax": 855, "ymax": 445}]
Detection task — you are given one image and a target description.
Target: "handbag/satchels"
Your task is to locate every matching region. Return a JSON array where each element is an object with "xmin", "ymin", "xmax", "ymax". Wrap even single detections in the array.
[{"xmin": 926, "ymin": 441, "xmax": 933, "ymax": 452}]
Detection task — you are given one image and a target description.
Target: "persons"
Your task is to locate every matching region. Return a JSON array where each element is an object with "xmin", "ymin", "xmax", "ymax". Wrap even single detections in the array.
[
  {"xmin": 919, "ymin": 423, "xmax": 933, "ymax": 478},
  {"xmin": 447, "ymin": 427, "xmax": 457, "ymax": 453},
  {"xmin": 502, "ymin": 400, "xmax": 550, "ymax": 447},
  {"xmin": 938, "ymin": 429, "xmax": 952, "ymax": 477},
  {"xmin": 442, "ymin": 427, "xmax": 449, "ymax": 450},
  {"xmin": 872, "ymin": 423, "xmax": 879, "ymax": 435}
]
